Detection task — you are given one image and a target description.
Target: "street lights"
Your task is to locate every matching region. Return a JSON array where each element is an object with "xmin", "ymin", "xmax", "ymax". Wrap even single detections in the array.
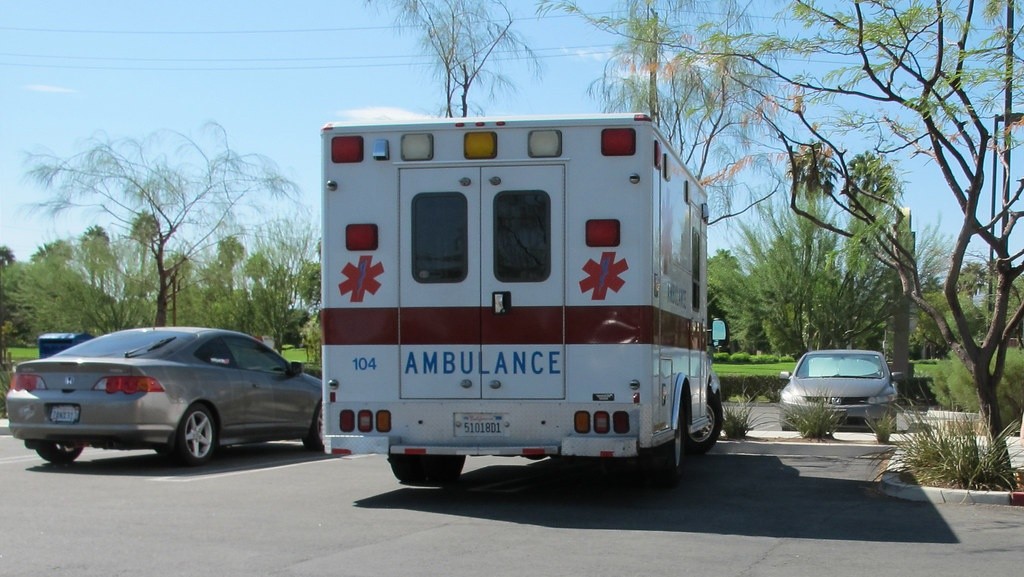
[{"xmin": 982, "ymin": 112, "xmax": 1022, "ymax": 332}]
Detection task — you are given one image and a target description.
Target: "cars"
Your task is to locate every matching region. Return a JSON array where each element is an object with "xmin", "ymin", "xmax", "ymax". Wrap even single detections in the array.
[
  {"xmin": 6, "ymin": 326, "xmax": 323, "ymax": 465},
  {"xmin": 777, "ymin": 350, "xmax": 903, "ymax": 434}
]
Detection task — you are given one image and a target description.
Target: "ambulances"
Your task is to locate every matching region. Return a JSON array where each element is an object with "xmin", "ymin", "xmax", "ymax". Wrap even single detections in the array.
[{"xmin": 317, "ymin": 111, "xmax": 730, "ymax": 487}]
